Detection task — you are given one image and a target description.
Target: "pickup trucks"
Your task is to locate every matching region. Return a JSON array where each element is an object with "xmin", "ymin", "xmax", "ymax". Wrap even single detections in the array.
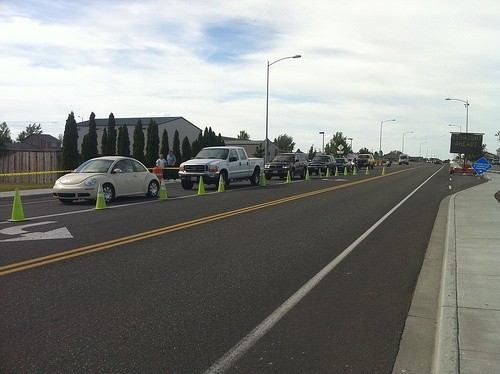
[{"xmin": 179, "ymin": 147, "xmax": 265, "ymax": 191}]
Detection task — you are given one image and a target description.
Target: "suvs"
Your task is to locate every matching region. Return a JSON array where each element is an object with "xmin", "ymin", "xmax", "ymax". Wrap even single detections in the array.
[{"xmin": 265, "ymin": 152, "xmax": 307, "ymax": 180}]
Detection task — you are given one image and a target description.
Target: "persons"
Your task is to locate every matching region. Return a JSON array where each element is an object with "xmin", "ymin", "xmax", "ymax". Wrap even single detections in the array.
[
  {"xmin": 166, "ymin": 150, "xmax": 176, "ymax": 180},
  {"xmin": 156, "ymin": 154, "xmax": 167, "ymax": 168}
]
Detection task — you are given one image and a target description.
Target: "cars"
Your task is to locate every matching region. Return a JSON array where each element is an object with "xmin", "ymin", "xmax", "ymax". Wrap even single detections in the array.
[
  {"xmin": 52, "ymin": 156, "xmax": 161, "ymax": 204},
  {"xmin": 307, "ymin": 154, "xmax": 376, "ymax": 176}
]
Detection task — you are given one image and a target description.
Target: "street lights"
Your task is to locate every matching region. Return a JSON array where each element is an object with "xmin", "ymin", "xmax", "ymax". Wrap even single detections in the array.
[
  {"xmin": 446, "ymin": 99, "xmax": 468, "ymax": 164},
  {"xmin": 348, "ymin": 138, "xmax": 353, "ymax": 148},
  {"xmin": 265, "ymin": 54, "xmax": 302, "ymax": 166},
  {"xmin": 380, "ymin": 119, "xmax": 396, "ymax": 158},
  {"xmin": 319, "ymin": 132, "xmax": 325, "ymax": 154},
  {"xmin": 402, "ymin": 131, "xmax": 414, "ymax": 153}
]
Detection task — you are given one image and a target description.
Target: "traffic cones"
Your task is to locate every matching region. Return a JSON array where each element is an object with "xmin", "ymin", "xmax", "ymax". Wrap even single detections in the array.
[
  {"xmin": 92, "ymin": 182, "xmax": 111, "ymax": 209},
  {"xmin": 158, "ymin": 178, "xmax": 171, "ymax": 200},
  {"xmin": 260, "ymin": 173, "xmax": 268, "ymax": 187},
  {"xmin": 216, "ymin": 174, "xmax": 227, "ymax": 192},
  {"xmin": 196, "ymin": 176, "xmax": 208, "ymax": 195},
  {"xmin": 305, "ymin": 169, "xmax": 311, "ymax": 181},
  {"xmin": 8, "ymin": 188, "xmax": 32, "ymax": 222},
  {"xmin": 318, "ymin": 166, "xmax": 385, "ymax": 178},
  {"xmin": 285, "ymin": 171, "xmax": 293, "ymax": 183}
]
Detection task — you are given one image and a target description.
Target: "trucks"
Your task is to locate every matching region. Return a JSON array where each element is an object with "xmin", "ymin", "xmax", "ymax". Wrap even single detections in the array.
[{"xmin": 399, "ymin": 154, "xmax": 410, "ymax": 165}]
